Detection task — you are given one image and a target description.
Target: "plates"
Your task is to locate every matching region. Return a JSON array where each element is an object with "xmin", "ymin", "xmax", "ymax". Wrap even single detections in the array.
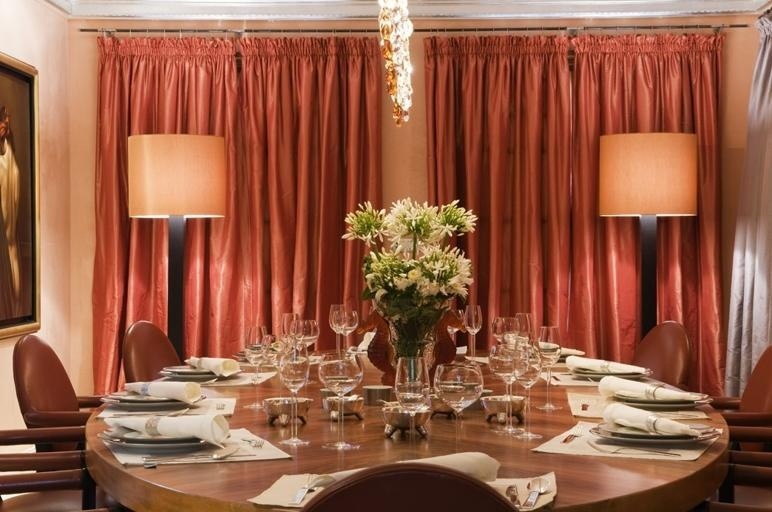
[
  {"xmin": 534, "ymin": 338, "xmax": 721, "ymax": 444},
  {"xmin": 97, "ymin": 360, "xmax": 242, "ymax": 452}
]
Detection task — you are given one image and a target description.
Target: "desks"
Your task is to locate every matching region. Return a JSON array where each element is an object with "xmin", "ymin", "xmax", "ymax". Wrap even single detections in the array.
[{"xmin": 83, "ymin": 345, "xmax": 732, "ymax": 512}]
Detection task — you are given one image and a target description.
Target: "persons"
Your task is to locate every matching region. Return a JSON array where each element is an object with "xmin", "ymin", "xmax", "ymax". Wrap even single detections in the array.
[{"xmin": 1, "ymin": 108, "xmax": 21, "ymax": 321}]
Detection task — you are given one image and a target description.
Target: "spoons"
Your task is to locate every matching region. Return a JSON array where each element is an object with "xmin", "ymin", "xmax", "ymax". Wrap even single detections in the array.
[{"xmin": 521, "ymin": 476, "xmax": 552, "ymax": 508}]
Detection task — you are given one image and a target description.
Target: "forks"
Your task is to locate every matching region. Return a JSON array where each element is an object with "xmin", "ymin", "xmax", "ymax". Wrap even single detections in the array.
[
  {"xmin": 291, "ymin": 472, "xmax": 335, "ymax": 504},
  {"xmin": 584, "ymin": 437, "xmax": 684, "ymax": 459},
  {"xmin": 506, "ymin": 483, "xmax": 520, "ymax": 507},
  {"xmin": 139, "ymin": 448, "xmax": 242, "ymax": 468}
]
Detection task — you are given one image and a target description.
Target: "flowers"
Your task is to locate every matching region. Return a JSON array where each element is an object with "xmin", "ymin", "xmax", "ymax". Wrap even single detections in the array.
[{"xmin": 342, "ymin": 197, "xmax": 479, "ymax": 393}]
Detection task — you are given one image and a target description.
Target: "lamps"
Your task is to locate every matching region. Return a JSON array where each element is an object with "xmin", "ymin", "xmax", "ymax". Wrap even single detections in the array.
[
  {"xmin": 378, "ymin": 0, "xmax": 414, "ymax": 128},
  {"xmin": 127, "ymin": 134, "xmax": 227, "ymax": 360},
  {"xmin": 598, "ymin": 132, "xmax": 699, "ymax": 339}
]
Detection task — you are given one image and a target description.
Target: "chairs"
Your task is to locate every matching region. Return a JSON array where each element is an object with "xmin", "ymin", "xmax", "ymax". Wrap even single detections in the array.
[
  {"xmin": 1, "ymin": 321, "xmax": 184, "ymax": 511},
  {"xmin": 628, "ymin": 321, "xmax": 770, "ymax": 510}
]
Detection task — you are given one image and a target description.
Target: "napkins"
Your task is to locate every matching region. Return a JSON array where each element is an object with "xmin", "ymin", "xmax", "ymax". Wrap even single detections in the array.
[
  {"xmin": 566, "ymin": 355, "xmax": 653, "ymax": 377},
  {"xmin": 185, "ymin": 355, "xmax": 241, "ymax": 377},
  {"xmin": 106, "ymin": 414, "xmax": 231, "ymax": 448},
  {"xmin": 303, "ymin": 451, "xmax": 501, "ymax": 490},
  {"xmin": 125, "ymin": 381, "xmax": 202, "ymax": 404},
  {"xmin": 602, "ymin": 402, "xmax": 699, "ymax": 439},
  {"xmin": 598, "ymin": 375, "xmax": 702, "ymax": 402}
]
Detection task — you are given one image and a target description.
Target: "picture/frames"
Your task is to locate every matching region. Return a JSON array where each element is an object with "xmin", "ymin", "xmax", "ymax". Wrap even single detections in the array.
[{"xmin": 0, "ymin": 51, "xmax": 41, "ymax": 340}]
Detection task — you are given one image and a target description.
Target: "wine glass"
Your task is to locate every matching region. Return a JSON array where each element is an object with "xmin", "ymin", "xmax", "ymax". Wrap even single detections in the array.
[
  {"xmin": 516, "ymin": 344, "xmax": 540, "ymax": 421},
  {"xmin": 434, "ymin": 363, "xmax": 483, "ymax": 452},
  {"xmin": 243, "ymin": 328, "xmax": 268, "ymax": 408},
  {"xmin": 464, "ymin": 305, "xmax": 481, "ymax": 362},
  {"xmin": 518, "ymin": 313, "xmax": 533, "ymax": 338},
  {"xmin": 321, "ymin": 351, "xmax": 358, "ymax": 450},
  {"xmin": 331, "ymin": 304, "xmax": 358, "ymax": 354},
  {"xmin": 493, "ymin": 316, "xmax": 520, "ymax": 343},
  {"xmin": 292, "ymin": 320, "xmax": 318, "ymax": 350},
  {"xmin": 277, "ymin": 314, "xmax": 303, "ymax": 353},
  {"xmin": 396, "ymin": 358, "xmax": 429, "ymax": 457},
  {"xmin": 537, "ymin": 325, "xmax": 560, "ymax": 411},
  {"xmin": 491, "ymin": 344, "xmax": 524, "ymax": 433},
  {"xmin": 280, "ymin": 344, "xmax": 309, "ymax": 447}
]
